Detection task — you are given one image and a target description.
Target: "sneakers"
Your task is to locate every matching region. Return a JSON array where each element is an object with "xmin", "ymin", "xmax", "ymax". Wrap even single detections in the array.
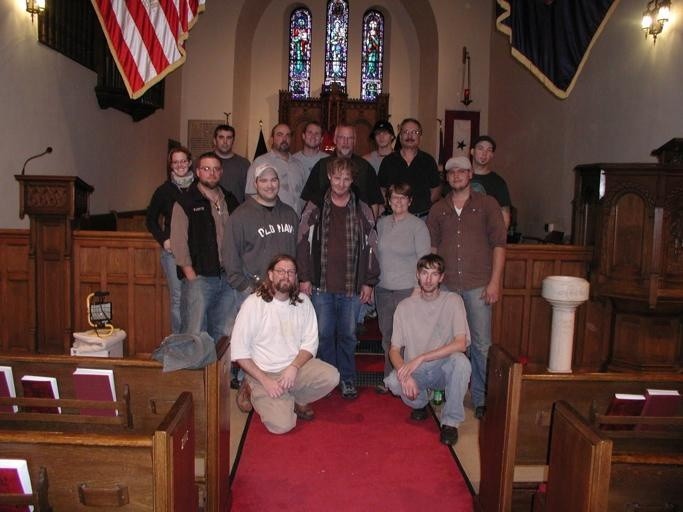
[
  {"xmin": 434, "ymin": 391, "xmax": 445, "ymax": 405},
  {"xmin": 476, "ymin": 406, "xmax": 484, "ymax": 419},
  {"xmin": 341, "ymin": 380, "xmax": 357, "ymax": 399},
  {"xmin": 411, "ymin": 407, "xmax": 427, "ymax": 421},
  {"xmin": 294, "ymin": 401, "xmax": 315, "ymax": 420},
  {"xmin": 440, "ymin": 424, "xmax": 458, "ymax": 445},
  {"xmin": 237, "ymin": 378, "xmax": 253, "ymax": 412}
]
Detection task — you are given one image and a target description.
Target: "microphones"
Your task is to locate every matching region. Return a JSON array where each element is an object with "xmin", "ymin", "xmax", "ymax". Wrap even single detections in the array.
[{"xmin": 22, "ymin": 146, "xmax": 53, "ymax": 174}]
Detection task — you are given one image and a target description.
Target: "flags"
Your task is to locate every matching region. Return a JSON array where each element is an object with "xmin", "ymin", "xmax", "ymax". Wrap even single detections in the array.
[{"xmin": 92, "ymin": 0, "xmax": 205, "ymax": 100}]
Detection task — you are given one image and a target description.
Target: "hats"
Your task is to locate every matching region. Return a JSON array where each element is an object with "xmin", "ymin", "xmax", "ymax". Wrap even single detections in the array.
[
  {"xmin": 254, "ymin": 162, "xmax": 279, "ymax": 179},
  {"xmin": 369, "ymin": 121, "xmax": 395, "ymax": 143},
  {"xmin": 446, "ymin": 157, "xmax": 471, "ymax": 171}
]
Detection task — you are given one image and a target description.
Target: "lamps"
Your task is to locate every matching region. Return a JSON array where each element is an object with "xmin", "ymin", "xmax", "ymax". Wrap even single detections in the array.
[{"xmin": 640, "ymin": 0, "xmax": 672, "ymax": 46}]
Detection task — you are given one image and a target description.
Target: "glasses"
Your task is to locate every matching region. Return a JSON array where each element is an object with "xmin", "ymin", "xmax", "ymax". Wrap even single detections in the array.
[
  {"xmin": 391, "ymin": 196, "xmax": 408, "ymax": 201},
  {"xmin": 171, "ymin": 160, "xmax": 189, "ymax": 163},
  {"xmin": 401, "ymin": 131, "xmax": 419, "ymax": 134},
  {"xmin": 272, "ymin": 270, "xmax": 297, "ymax": 276},
  {"xmin": 200, "ymin": 167, "xmax": 222, "ymax": 171}
]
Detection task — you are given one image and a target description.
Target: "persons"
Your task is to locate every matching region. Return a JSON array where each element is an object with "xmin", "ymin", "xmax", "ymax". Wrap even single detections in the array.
[
  {"xmin": 143, "ymin": 117, "xmax": 514, "ymax": 446},
  {"xmin": 366, "ymin": 19, "xmax": 379, "ymax": 79},
  {"xmin": 330, "ymin": 17, "xmax": 343, "ymax": 74}
]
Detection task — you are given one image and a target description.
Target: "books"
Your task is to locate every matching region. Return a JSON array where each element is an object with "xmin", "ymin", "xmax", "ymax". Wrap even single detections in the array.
[
  {"xmin": 20, "ymin": 374, "xmax": 63, "ymax": 418},
  {"xmin": 72, "ymin": 366, "xmax": 120, "ymax": 417},
  {"xmin": 1, "ymin": 452, "xmax": 35, "ymax": 512},
  {"xmin": 635, "ymin": 388, "xmax": 681, "ymax": 435},
  {"xmin": 597, "ymin": 393, "xmax": 647, "ymax": 440},
  {"xmin": 1, "ymin": 361, "xmax": 19, "ymax": 415}
]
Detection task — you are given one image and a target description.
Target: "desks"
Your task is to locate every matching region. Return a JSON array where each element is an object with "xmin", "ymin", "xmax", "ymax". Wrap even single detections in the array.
[
  {"xmin": 480, "ymin": 343, "xmax": 682, "ymax": 512},
  {"xmin": 0, "ymin": 346, "xmax": 233, "ymax": 511}
]
